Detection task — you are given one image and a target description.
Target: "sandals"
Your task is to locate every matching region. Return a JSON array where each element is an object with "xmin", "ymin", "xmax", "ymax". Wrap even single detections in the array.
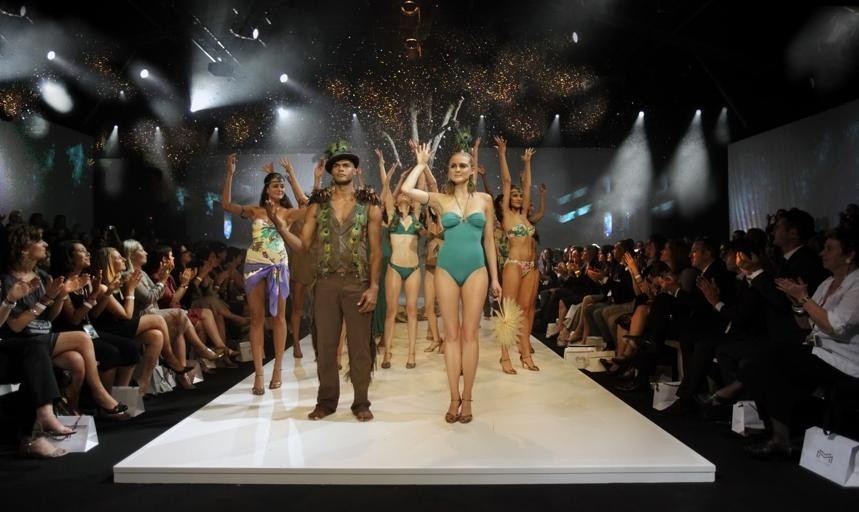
[
  {"xmin": 18, "ymin": 438, "xmax": 69, "ymax": 458},
  {"xmin": 32, "ymin": 415, "xmax": 76, "ymax": 436}
]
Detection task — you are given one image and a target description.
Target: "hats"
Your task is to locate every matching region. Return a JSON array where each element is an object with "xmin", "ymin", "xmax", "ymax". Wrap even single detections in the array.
[{"xmin": 324, "ymin": 142, "xmax": 359, "ymax": 172}]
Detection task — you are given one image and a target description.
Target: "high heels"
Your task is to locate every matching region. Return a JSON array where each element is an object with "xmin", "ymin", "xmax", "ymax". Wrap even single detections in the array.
[
  {"xmin": 167, "ymin": 348, "xmax": 241, "ymax": 390},
  {"xmin": 520, "ymin": 355, "xmax": 539, "ymax": 371},
  {"xmin": 500, "ymin": 357, "xmax": 516, "ymax": 374},
  {"xmin": 459, "ymin": 397, "xmax": 473, "ymax": 423},
  {"xmin": 253, "ymin": 374, "xmax": 265, "ymax": 395},
  {"xmin": 293, "ymin": 344, "xmax": 303, "ymax": 358},
  {"xmin": 270, "ymin": 370, "xmax": 281, "ymax": 389},
  {"xmin": 91, "ymin": 396, "xmax": 128, "ymax": 415},
  {"xmin": 445, "ymin": 397, "xmax": 461, "ymax": 423},
  {"xmin": 405, "ymin": 353, "xmax": 416, "ymax": 369},
  {"xmin": 424, "ymin": 338, "xmax": 442, "ymax": 353},
  {"xmin": 382, "ymin": 353, "xmax": 391, "ymax": 368}
]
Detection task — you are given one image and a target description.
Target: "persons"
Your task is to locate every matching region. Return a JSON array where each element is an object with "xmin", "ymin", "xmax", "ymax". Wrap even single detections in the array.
[
  {"xmin": 1, "ymin": 211, "xmax": 294, "ymax": 441},
  {"xmin": 527, "ymin": 205, "xmax": 858, "ymax": 456},
  {"xmin": 222, "ymin": 151, "xmax": 326, "ymax": 395},
  {"xmin": 265, "ymin": 141, "xmax": 382, "ymax": 422},
  {"xmin": 400, "ymin": 142, "xmax": 502, "ymax": 424},
  {"xmin": 261, "ymin": 143, "xmax": 547, "ymax": 378},
  {"xmin": 494, "ymin": 134, "xmax": 539, "ymax": 374}
]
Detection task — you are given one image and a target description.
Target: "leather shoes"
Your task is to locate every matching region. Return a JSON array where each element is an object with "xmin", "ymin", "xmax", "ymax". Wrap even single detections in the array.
[
  {"xmin": 600, "ymin": 335, "xmax": 650, "ymax": 390},
  {"xmin": 308, "ymin": 408, "xmax": 327, "ymax": 420},
  {"xmin": 692, "ymin": 390, "xmax": 727, "ymax": 407},
  {"xmin": 354, "ymin": 409, "xmax": 373, "ymax": 422}
]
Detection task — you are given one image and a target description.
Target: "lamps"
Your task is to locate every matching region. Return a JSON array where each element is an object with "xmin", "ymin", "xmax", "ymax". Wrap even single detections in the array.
[{"xmin": 227, "ymin": 7, "xmax": 259, "ymax": 39}]
